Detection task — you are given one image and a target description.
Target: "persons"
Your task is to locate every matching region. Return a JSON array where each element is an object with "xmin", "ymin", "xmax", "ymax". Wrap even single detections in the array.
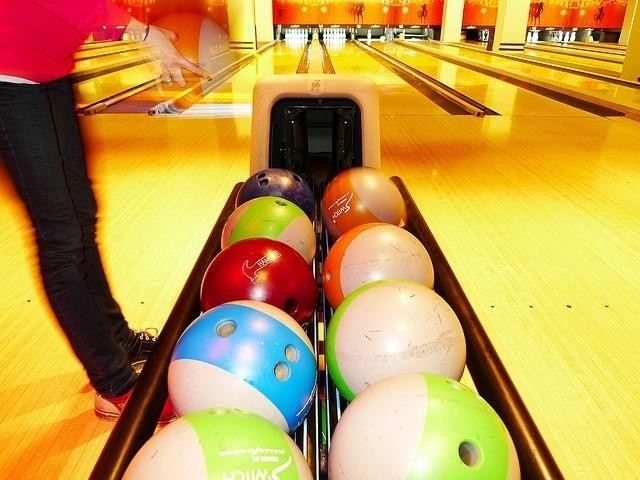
[{"xmin": 0, "ymin": 0, "xmax": 213, "ymax": 425}]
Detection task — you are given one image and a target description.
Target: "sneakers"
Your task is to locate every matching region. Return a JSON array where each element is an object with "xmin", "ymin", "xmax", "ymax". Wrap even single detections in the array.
[
  {"xmin": 127, "ymin": 327, "xmax": 160, "ymax": 366},
  {"xmin": 94, "ymin": 386, "xmax": 178, "ymax": 425}
]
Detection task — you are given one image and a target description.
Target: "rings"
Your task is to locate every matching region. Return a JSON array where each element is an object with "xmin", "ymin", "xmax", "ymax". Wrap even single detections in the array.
[{"xmin": 168, "ymin": 78, "xmax": 171, "ymax": 82}]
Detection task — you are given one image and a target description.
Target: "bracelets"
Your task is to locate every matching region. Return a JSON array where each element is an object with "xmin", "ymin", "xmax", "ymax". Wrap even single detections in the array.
[{"xmin": 143, "ymin": 24, "xmax": 149, "ymax": 41}]
